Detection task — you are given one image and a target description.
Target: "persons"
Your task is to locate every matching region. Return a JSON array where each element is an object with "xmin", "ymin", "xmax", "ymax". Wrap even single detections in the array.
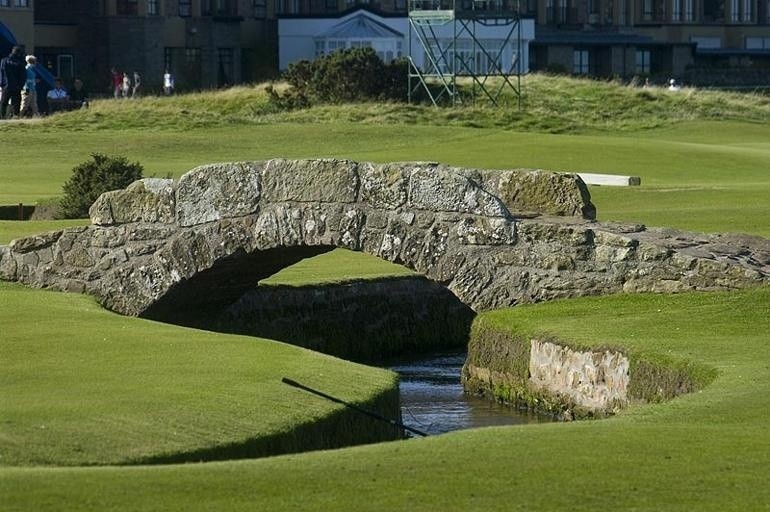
[
  {"xmin": 643, "ymin": 77, "xmax": 681, "ymax": 92},
  {"xmin": 108, "ymin": 67, "xmax": 142, "ymax": 99},
  {"xmin": 0, "ymin": 44, "xmax": 89, "ymax": 120},
  {"xmin": 162, "ymin": 67, "xmax": 175, "ymax": 96}
]
